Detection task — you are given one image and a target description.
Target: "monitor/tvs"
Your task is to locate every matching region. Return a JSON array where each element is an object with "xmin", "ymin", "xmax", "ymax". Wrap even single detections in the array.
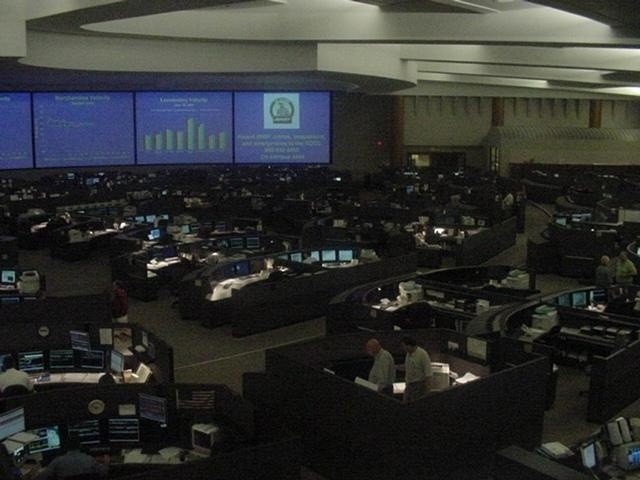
[
  {"xmin": 27, "ymin": 425, "xmax": 62, "ymax": 454},
  {"xmin": 290, "ymin": 252, "xmax": 302, "ymax": 263},
  {"xmin": 558, "ymin": 293, "xmax": 571, "ymax": 307},
  {"xmin": 161, "ymin": 214, "xmax": 168, "ymax": 220},
  {"xmin": 80, "ymin": 350, "xmax": 104, "ymax": 369},
  {"xmin": 48, "ymin": 348, "xmax": 76, "ymax": 369},
  {"xmin": 590, "ymin": 289, "xmax": 606, "ymax": 304},
  {"xmin": 321, "ymin": 250, "xmax": 336, "ymax": 261},
  {"xmin": 215, "ymin": 221, "xmax": 226, "ymax": 231},
  {"xmin": 278, "ymin": 255, "xmax": 288, "ymax": 261},
  {"xmin": 230, "ymin": 237, "xmax": 245, "ymax": 250},
  {"xmin": 0, "ymin": 353, "xmax": 12, "ymax": 372},
  {"xmin": 189, "ymin": 423, "xmax": 219, "ymax": 458},
  {"xmin": 235, "ymin": 260, "xmax": 250, "ymax": 275},
  {"xmin": 93, "ymin": 177, "xmax": 99, "ymax": 183},
  {"xmin": 86, "ymin": 178, "xmax": 93, "ymax": 185},
  {"xmin": 135, "ymin": 216, "xmax": 144, "ymax": 223},
  {"xmin": 616, "ymin": 441, "xmax": 640, "ymax": 470},
  {"xmin": 1, "ymin": 270, "xmax": 16, "ymax": 283},
  {"xmin": 246, "ymin": 236, "xmax": 261, "ymax": 249},
  {"xmin": 16, "ymin": 350, "xmax": 45, "ymax": 373},
  {"xmin": 0, "ymin": 406, "xmax": 25, "ymax": 442},
  {"xmin": 203, "ymin": 222, "xmax": 213, "ymax": 231},
  {"xmin": 572, "ymin": 291, "xmax": 587, "ymax": 309},
  {"xmin": 466, "ymin": 335, "xmax": 488, "ymax": 366},
  {"xmin": 146, "ymin": 214, "xmax": 156, "ymax": 223},
  {"xmin": 338, "ymin": 250, "xmax": 353, "ymax": 261},
  {"xmin": 68, "ymin": 173, "xmax": 75, "ymax": 180},
  {"xmin": 110, "ymin": 350, "xmax": 124, "ymax": 373},
  {"xmin": 151, "ymin": 228, "xmax": 161, "ymax": 239},
  {"xmin": 138, "ymin": 392, "xmax": 167, "ymax": 423},
  {"xmin": 108, "ymin": 418, "xmax": 139, "ymax": 442},
  {"xmin": 67, "ymin": 419, "xmax": 100, "ymax": 446},
  {"xmin": 69, "ymin": 330, "xmax": 91, "ymax": 352},
  {"xmin": 180, "ymin": 224, "xmax": 192, "ymax": 234},
  {"xmin": 580, "ymin": 440, "xmax": 598, "ymax": 469},
  {"xmin": 311, "ymin": 251, "xmax": 319, "ymax": 261}
]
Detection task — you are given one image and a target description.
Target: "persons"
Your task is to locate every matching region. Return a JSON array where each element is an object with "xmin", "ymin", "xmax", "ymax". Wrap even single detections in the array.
[
  {"xmin": 109, "ymin": 279, "xmax": 128, "ymax": 324},
  {"xmin": 595, "ymin": 255, "xmax": 614, "ymax": 285},
  {"xmin": 0, "ymin": 355, "xmax": 38, "ymax": 396},
  {"xmin": 364, "ymin": 337, "xmax": 397, "ymax": 398},
  {"xmin": 302, "ymin": 249, "xmax": 318, "ymax": 264},
  {"xmin": 615, "ymin": 250, "xmax": 638, "ymax": 286},
  {"xmin": 32, "ymin": 430, "xmax": 112, "ymax": 480},
  {"xmin": 397, "ymin": 335, "xmax": 434, "ymax": 403},
  {"xmin": 206, "ymin": 252, "xmax": 220, "ymax": 265}
]
